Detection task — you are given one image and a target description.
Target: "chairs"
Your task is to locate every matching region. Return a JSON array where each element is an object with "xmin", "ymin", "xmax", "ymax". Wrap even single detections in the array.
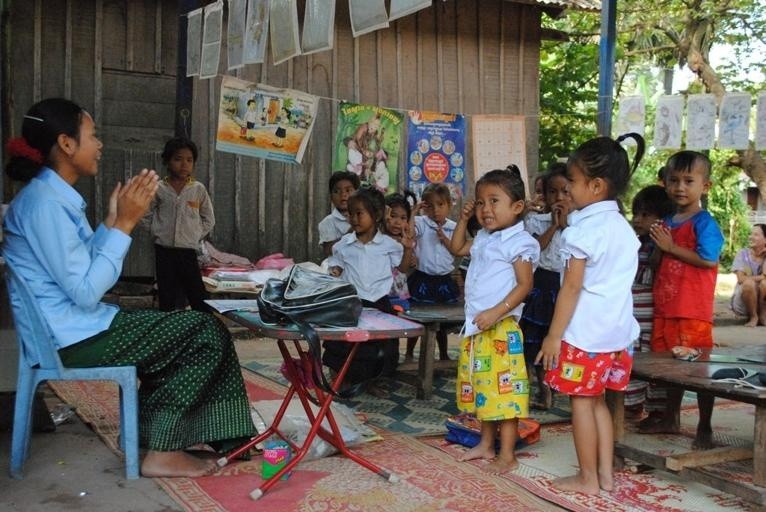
[{"xmin": 1, "ymin": 250, "xmax": 139, "ymax": 480}]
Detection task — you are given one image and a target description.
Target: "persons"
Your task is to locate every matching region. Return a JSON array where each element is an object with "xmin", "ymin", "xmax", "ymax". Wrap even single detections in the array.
[
  {"xmin": 317, "ymin": 172, "xmax": 464, "ymax": 399},
  {"xmin": 240, "ymin": 99, "xmax": 391, "ymax": 194},
  {"xmin": 134, "ymin": 138, "xmax": 215, "ymax": 314},
  {"xmin": 1, "ymin": 98, "xmax": 259, "ymax": 479},
  {"xmin": 449, "ymin": 131, "xmax": 726, "ymax": 495},
  {"xmin": 729, "ymin": 223, "xmax": 766, "ymax": 328}
]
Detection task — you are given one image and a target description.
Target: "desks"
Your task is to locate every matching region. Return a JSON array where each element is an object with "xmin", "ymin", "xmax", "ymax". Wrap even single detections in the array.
[
  {"xmin": 203, "ymin": 299, "xmax": 424, "ymax": 500},
  {"xmin": 394, "ymin": 306, "xmax": 465, "ymax": 399}
]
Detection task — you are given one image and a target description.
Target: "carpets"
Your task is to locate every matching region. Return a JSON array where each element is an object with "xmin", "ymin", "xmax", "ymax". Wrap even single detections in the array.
[{"xmin": 45, "ymin": 346, "xmax": 766, "ymax": 512}]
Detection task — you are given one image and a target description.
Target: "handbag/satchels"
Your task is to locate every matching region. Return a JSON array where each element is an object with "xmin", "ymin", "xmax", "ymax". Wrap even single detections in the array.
[
  {"xmin": 257, "ymin": 264, "xmax": 363, "ymax": 327},
  {"xmin": 445, "ymin": 413, "xmax": 540, "ymax": 454}
]
